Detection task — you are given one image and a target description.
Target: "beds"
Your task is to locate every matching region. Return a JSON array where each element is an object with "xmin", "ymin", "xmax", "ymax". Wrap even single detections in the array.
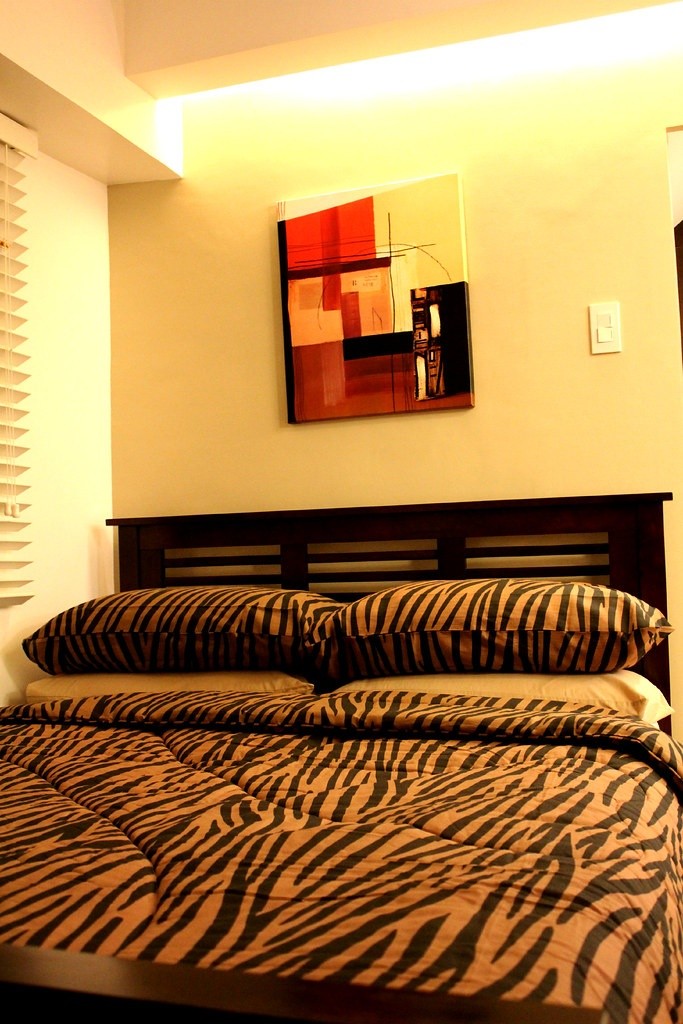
[{"xmin": 0, "ymin": 492, "xmax": 683, "ymax": 1024}]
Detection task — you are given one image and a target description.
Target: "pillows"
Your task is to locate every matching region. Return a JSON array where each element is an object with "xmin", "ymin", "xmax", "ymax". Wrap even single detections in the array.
[
  {"xmin": 21, "ymin": 586, "xmax": 344, "ymax": 676},
  {"xmin": 305, "ymin": 579, "xmax": 675, "ymax": 686},
  {"xmin": 26, "ymin": 669, "xmax": 317, "ymax": 703},
  {"xmin": 331, "ymin": 668, "xmax": 676, "ymax": 722}
]
